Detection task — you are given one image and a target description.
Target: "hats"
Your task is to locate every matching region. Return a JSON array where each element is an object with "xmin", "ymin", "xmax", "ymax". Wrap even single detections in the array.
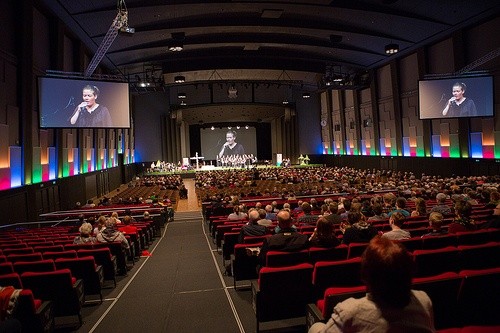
[{"xmin": 79, "ymin": 223, "xmax": 93, "ymax": 234}]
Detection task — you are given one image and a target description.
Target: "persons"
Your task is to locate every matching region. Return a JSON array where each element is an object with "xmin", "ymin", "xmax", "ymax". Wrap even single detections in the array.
[
  {"xmin": 441, "ymin": 82, "xmax": 478, "ymax": 117},
  {"xmin": 151, "ymin": 160, "xmax": 181, "ymax": 170},
  {"xmin": 67, "ymin": 85, "xmax": 112, "ymax": 127},
  {"xmin": 309, "ymin": 238, "xmax": 435, "ymax": 333},
  {"xmin": 129, "ymin": 174, "xmax": 187, "ymax": 199},
  {"xmin": 220, "ymin": 130, "xmax": 245, "ymax": 159},
  {"xmin": 69, "ymin": 211, "xmax": 137, "ymax": 250},
  {"xmin": 282, "ymin": 158, "xmax": 290, "ymax": 166},
  {"xmin": 222, "ymin": 154, "xmax": 257, "ymax": 169},
  {"xmin": 73, "ymin": 192, "xmax": 171, "ymax": 210},
  {"xmin": 196, "ymin": 165, "xmax": 500, "ymax": 262},
  {"xmin": 144, "ymin": 211, "xmax": 153, "ymax": 221}
]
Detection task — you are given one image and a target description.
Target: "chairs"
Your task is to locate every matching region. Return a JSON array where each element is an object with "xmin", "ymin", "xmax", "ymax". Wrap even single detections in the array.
[
  {"xmin": 0, "ymin": 222, "xmax": 153, "ymax": 333},
  {"xmin": 206, "ymin": 204, "xmax": 500, "ymax": 333}
]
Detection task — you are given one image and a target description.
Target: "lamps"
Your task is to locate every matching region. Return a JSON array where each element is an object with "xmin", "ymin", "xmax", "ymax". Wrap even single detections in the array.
[
  {"xmin": 385, "ymin": 44, "xmax": 399, "ymax": 54},
  {"xmin": 177, "ymin": 93, "xmax": 186, "ymax": 98},
  {"xmin": 302, "ymin": 93, "xmax": 311, "ymax": 99},
  {"xmin": 174, "ymin": 76, "xmax": 185, "ymax": 83},
  {"xmin": 121, "ymin": 27, "xmax": 135, "ymax": 33},
  {"xmin": 169, "ymin": 40, "xmax": 183, "ymax": 53}
]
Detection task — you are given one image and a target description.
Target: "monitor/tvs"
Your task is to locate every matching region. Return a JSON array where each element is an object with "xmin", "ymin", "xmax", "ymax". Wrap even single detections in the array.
[
  {"xmin": 33, "ymin": 76, "xmax": 130, "ymax": 131},
  {"xmin": 417, "ymin": 75, "xmax": 495, "ymax": 120}
]
